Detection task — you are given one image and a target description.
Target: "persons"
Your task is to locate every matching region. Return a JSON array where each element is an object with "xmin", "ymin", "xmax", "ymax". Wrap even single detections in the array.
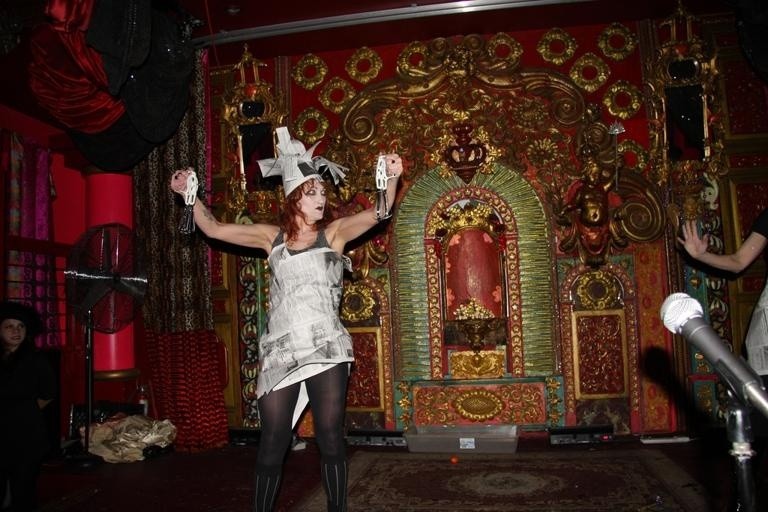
[
  {"xmin": 555, "ymin": 155, "xmax": 628, "ymax": 259},
  {"xmin": 171, "ymin": 151, "xmax": 405, "ymax": 511},
  {"xmin": 675, "ymin": 189, "xmax": 768, "ymax": 378},
  {"xmin": 0, "ymin": 300, "xmax": 61, "ymax": 511}
]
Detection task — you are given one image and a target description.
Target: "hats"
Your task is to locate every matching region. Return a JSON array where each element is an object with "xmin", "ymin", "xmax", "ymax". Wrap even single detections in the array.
[{"xmin": 0, "ymin": 301, "xmax": 42, "ymax": 336}]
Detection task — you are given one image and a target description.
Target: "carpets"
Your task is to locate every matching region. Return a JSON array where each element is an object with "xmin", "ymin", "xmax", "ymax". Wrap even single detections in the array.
[{"xmin": 287, "ymin": 445, "xmax": 732, "ymax": 512}]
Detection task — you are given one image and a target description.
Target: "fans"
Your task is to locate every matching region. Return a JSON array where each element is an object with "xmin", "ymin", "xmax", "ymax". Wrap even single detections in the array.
[{"xmin": 64, "ymin": 222, "xmax": 154, "ymax": 473}]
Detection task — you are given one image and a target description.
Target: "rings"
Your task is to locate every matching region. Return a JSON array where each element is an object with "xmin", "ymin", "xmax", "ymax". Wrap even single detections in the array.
[
  {"xmin": 175, "ymin": 175, "xmax": 179, "ymax": 179},
  {"xmin": 178, "ymin": 172, "xmax": 182, "ymax": 176},
  {"xmin": 391, "ymin": 158, "xmax": 396, "ymax": 164}
]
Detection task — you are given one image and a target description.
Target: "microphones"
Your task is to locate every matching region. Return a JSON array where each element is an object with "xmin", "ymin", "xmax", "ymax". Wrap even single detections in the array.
[{"xmin": 660, "ymin": 291, "xmax": 768, "ymax": 418}]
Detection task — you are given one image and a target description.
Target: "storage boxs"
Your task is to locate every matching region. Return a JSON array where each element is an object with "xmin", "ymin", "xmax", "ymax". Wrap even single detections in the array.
[{"xmin": 404, "ymin": 424, "xmax": 517, "ymax": 453}]
